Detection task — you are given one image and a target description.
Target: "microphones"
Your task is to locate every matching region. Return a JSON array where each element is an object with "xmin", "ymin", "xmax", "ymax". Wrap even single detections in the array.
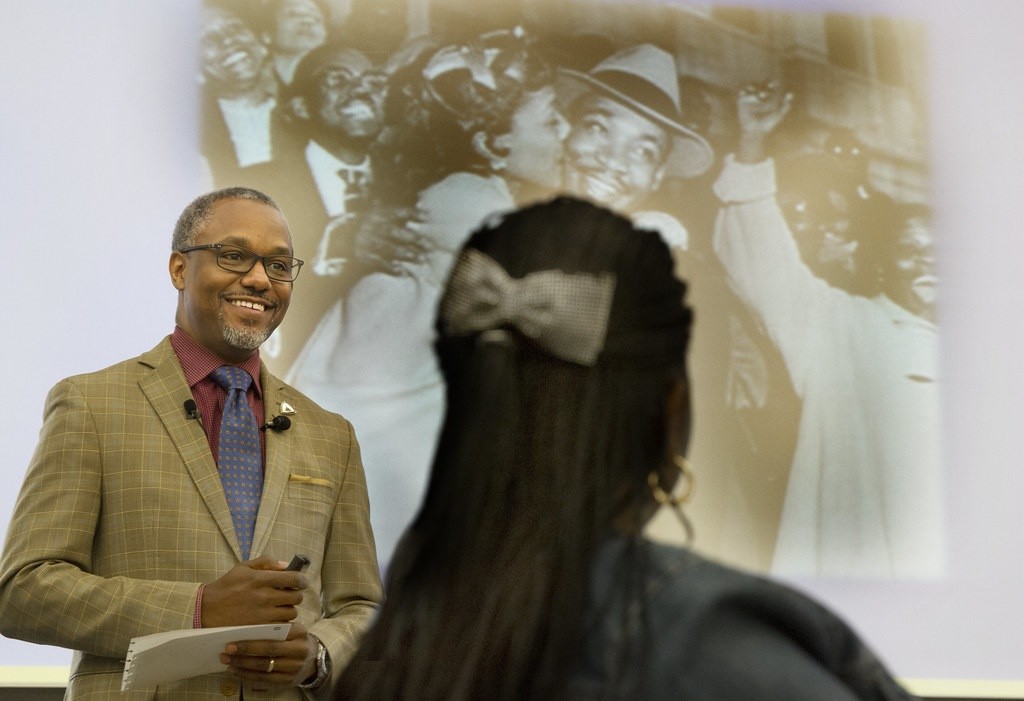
[
  {"xmin": 266, "ymin": 415, "xmax": 291, "ymax": 431},
  {"xmin": 183, "ymin": 399, "xmax": 197, "ymax": 419}
]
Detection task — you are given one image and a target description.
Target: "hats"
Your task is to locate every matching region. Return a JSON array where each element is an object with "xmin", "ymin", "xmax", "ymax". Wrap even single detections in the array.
[{"xmin": 557, "ymin": 44, "xmax": 714, "ymax": 177}]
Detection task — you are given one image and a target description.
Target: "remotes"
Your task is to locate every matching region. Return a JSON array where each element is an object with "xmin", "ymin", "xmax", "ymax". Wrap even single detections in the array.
[{"xmin": 280, "ymin": 554, "xmax": 311, "ymax": 591}]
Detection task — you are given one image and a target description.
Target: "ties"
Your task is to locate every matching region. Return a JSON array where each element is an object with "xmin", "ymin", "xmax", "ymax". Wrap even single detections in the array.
[
  {"xmin": 339, "ymin": 170, "xmax": 366, "ymax": 213},
  {"xmin": 210, "ymin": 366, "xmax": 264, "ymax": 561}
]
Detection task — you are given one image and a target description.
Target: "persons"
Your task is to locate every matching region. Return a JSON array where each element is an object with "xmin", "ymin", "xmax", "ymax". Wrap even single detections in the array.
[
  {"xmin": 195, "ymin": 3, "xmax": 947, "ymax": 575},
  {"xmin": 326, "ymin": 193, "xmax": 913, "ymax": 700},
  {"xmin": 0, "ymin": 185, "xmax": 386, "ymax": 701}
]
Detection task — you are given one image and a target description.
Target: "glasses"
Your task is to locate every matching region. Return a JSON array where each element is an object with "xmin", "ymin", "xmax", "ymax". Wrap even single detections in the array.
[{"xmin": 180, "ymin": 243, "xmax": 303, "ymax": 282}]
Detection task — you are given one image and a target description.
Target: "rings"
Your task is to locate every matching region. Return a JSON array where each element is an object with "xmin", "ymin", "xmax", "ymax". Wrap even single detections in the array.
[{"xmin": 267, "ymin": 658, "xmax": 275, "ymax": 672}]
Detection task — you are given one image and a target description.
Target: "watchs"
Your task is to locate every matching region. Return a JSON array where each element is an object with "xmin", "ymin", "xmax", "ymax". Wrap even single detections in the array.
[{"xmin": 296, "ymin": 634, "xmax": 330, "ymax": 689}]
